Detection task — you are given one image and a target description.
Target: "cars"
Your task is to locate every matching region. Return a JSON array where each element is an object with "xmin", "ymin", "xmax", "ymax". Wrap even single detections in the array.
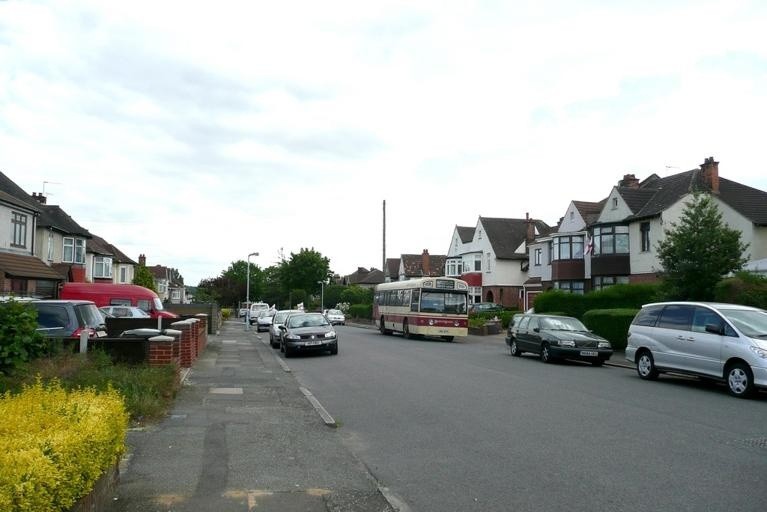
[
  {"xmin": 0, "ymin": 294, "xmax": 150, "ymax": 340},
  {"xmin": 504, "ymin": 313, "xmax": 614, "ymax": 367},
  {"xmin": 238, "ymin": 302, "xmax": 346, "ymax": 358}
]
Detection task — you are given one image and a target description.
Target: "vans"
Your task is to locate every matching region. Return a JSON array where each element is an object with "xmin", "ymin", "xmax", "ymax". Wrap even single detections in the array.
[
  {"xmin": 626, "ymin": 302, "xmax": 767, "ymax": 400},
  {"xmin": 58, "ymin": 281, "xmax": 180, "ymax": 320}
]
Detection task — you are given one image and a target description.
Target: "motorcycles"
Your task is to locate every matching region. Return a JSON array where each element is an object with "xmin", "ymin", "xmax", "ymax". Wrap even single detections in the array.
[
  {"xmin": 317, "ymin": 280, "xmax": 323, "ymax": 314},
  {"xmin": 245, "ymin": 252, "xmax": 259, "ymax": 332}
]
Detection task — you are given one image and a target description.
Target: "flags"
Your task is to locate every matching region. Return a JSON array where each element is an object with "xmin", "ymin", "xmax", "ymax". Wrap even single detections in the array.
[{"xmin": 583, "ymin": 236, "xmax": 594, "ymax": 256}]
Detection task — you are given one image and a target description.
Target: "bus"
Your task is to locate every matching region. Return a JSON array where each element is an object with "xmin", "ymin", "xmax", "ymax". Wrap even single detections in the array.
[{"xmin": 373, "ymin": 276, "xmax": 469, "ymax": 343}]
